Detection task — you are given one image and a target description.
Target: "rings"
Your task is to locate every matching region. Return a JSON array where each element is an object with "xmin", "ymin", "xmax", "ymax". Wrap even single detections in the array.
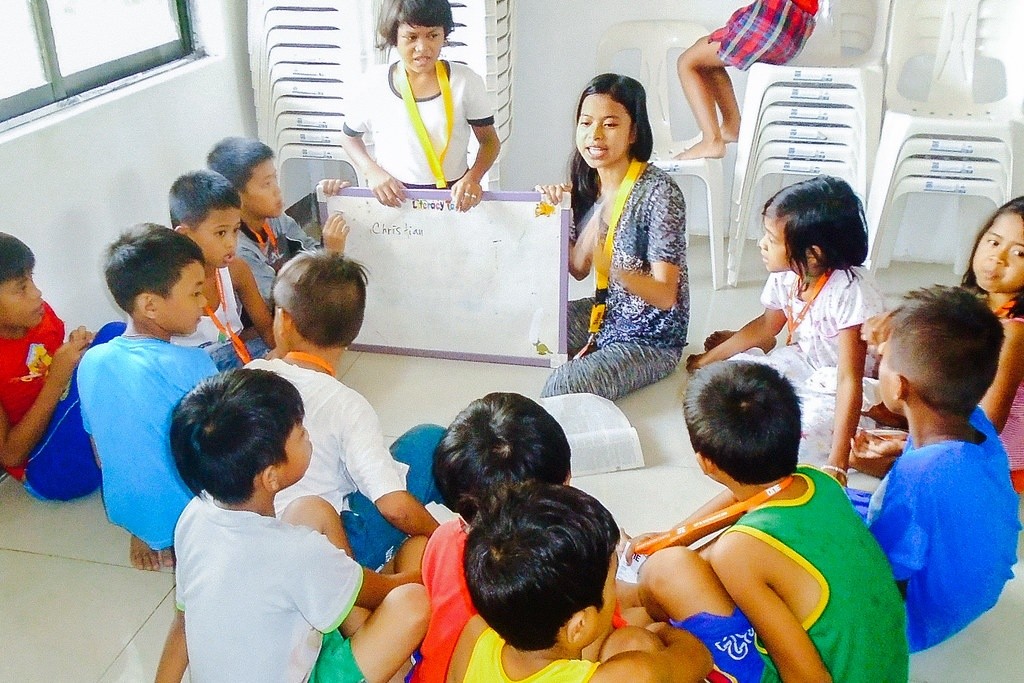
[
  {"xmin": 470, "ymin": 195, "xmax": 476, "ymax": 199},
  {"xmin": 464, "ymin": 193, "xmax": 470, "ymax": 197}
]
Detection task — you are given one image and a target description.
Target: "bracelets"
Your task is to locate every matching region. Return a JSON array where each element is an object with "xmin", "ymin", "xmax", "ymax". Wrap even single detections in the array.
[{"xmin": 821, "ymin": 464, "xmax": 849, "ymax": 481}]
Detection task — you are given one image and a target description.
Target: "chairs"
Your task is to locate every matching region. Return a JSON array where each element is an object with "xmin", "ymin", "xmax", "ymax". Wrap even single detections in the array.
[
  {"xmin": 597, "ymin": 0, "xmax": 1024, "ymax": 291},
  {"xmin": 246, "ymin": 0, "xmax": 512, "ymax": 191}
]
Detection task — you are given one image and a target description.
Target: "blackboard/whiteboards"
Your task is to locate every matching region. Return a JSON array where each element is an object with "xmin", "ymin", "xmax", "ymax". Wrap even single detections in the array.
[{"xmin": 315, "ymin": 184, "xmax": 570, "ymax": 367}]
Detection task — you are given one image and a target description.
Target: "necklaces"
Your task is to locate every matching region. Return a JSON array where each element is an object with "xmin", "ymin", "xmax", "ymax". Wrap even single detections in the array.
[{"xmin": 122, "ymin": 333, "xmax": 155, "ymax": 338}]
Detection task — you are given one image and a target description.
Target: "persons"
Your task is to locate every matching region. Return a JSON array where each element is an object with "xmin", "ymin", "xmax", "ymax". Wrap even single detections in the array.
[
  {"xmin": 341, "ymin": 0, "xmax": 501, "ymax": 212},
  {"xmin": 0, "ymin": 138, "xmax": 1024, "ymax": 683},
  {"xmin": 534, "ymin": 73, "xmax": 690, "ymax": 401},
  {"xmin": 676, "ymin": 0, "xmax": 819, "ymax": 159}
]
